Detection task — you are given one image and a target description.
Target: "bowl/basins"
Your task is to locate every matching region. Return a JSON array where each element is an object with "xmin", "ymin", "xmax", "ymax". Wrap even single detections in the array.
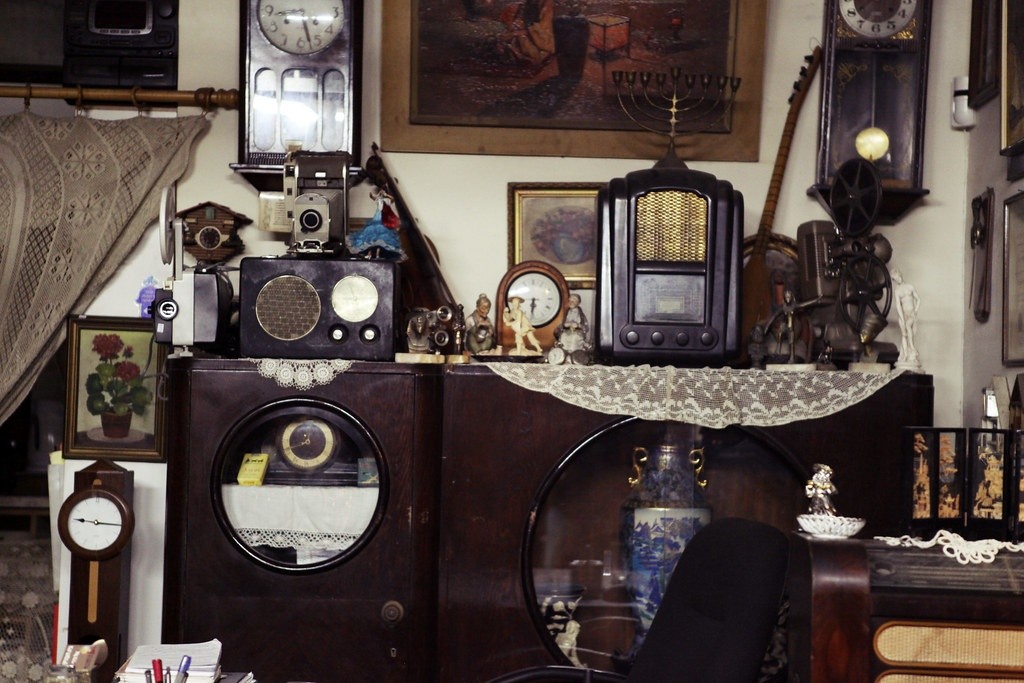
[{"xmin": 797, "ymin": 514, "xmax": 868, "ymax": 539}]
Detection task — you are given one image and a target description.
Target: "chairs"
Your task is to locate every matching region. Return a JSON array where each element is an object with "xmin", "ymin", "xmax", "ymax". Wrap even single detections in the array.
[{"xmin": 479, "ymin": 515, "xmax": 791, "ymax": 683}]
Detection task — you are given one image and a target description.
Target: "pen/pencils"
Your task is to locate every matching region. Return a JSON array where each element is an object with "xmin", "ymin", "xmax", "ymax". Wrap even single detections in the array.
[{"xmin": 145, "ymin": 655, "xmax": 191, "ymax": 683}]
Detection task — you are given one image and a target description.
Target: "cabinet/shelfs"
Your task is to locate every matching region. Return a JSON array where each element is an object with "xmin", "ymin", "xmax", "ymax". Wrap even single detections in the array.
[
  {"xmin": 433, "ymin": 359, "xmax": 934, "ymax": 683},
  {"xmin": 786, "ymin": 530, "xmax": 1024, "ymax": 683},
  {"xmin": 160, "ymin": 354, "xmax": 437, "ymax": 683}
]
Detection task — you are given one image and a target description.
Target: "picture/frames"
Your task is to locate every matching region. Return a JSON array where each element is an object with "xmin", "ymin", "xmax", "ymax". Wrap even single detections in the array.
[
  {"xmin": 379, "ymin": 0, "xmax": 766, "ymax": 164},
  {"xmin": 1000, "ymin": 0, "xmax": 1024, "ymax": 157},
  {"xmin": 506, "ymin": 180, "xmax": 600, "ymax": 290},
  {"xmin": 1001, "ymin": 192, "xmax": 1024, "ymax": 368},
  {"xmin": 968, "ymin": 0, "xmax": 1001, "ymax": 108},
  {"xmin": 61, "ymin": 316, "xmax": 168, "ymax": 463}
]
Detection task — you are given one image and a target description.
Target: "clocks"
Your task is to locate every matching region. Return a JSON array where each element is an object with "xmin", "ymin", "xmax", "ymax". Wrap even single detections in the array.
[
  {"xmin": 230, "ymin": 0, "xmax": 366, "ymax": 198},
  {"xmin": 496, "ymin": 260, "xmax": 572, "ymax": 349},
  {"xmin": 808, "ymin": 0, "xmax": 937, "ymax": 228},
  {"xmin": 276, "ymin": 416, "xmax": 342, "ymax": 477},
  {"xmin": 56, "ymin": 459, "xmax": 135, "ymax": 683}
]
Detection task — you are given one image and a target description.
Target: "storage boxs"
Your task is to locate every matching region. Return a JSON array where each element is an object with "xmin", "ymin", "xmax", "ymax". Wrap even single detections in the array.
[
  {"xmin": 238, "ymin": 453, "xmax": 269, "ymax": 487},
  {"xmin": 355, "ymin": 457, "xmax": 379, "ymax": 488}
]
